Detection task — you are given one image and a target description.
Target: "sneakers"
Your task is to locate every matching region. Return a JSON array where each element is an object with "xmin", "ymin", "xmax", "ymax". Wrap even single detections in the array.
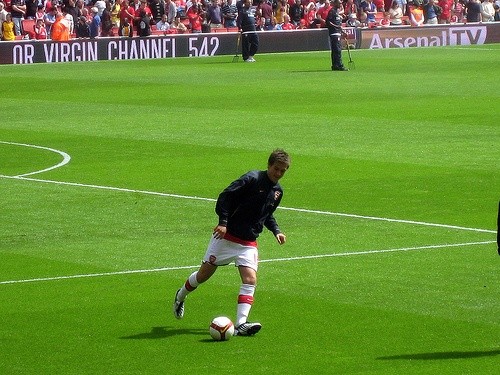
[
  {"xmin": 174, "ymin": 289, "xmax": 185, "ymax": 319},
  {"xmin": 332, "ymin": 66, "xmax": 340, "ymax": 70},
  {"xmin": 234, "ymin": 323, "xmax": 262, "ymax": 336},
  {"xmin": 249, "ymin": 57, "xmax": 256, "ymax": 62},
  {"xmin": 245, "ymin": 59, "xmax": 252, "ymax": 62},
  {"xmin": 338, "ymin": 67, "xmax": 349, "ymax": 71}
]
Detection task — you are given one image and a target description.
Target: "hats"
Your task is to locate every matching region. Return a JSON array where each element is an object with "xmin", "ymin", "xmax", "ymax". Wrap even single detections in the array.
[
  {"xmin": 91, "ymin": 7, "xmax": 98, "ymax": 13},
  {"xmin": 301, "ymin": 19, "xmax": 307, "ymax": 25},
  {"xmin": 349, "ymin": 13, "xmax": 358, "ymax": 19}
]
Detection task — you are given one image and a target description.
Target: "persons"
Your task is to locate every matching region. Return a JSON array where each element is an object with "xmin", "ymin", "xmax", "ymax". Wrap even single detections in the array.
[
  {"xmin": 0, "ymin": 0, "xmax": 500, "ymax": 40},
  {"xmin": 325, "ymin": 0, "xmax": 350, "ymax": 71},
  {"xmin": 236, "ymin": 0, "xmax": 259, "ymax": 63},
  {"xmin": 174, "ymin": 146, "xmax": 290, "ymax": 335}
]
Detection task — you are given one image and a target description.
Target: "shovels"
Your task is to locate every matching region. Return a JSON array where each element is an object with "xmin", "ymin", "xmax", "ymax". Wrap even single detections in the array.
[
  {"xmin": 343, "ymin": 38, "xmax": 355, "ymax": 71},
  {"xmin": 232, "ymin": 31, "xmax": 240, "ymax": 63}
]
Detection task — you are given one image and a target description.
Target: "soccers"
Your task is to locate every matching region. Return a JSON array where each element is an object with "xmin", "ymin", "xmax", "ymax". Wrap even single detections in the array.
[{"xmin": 209, "ymin": 316, "xmax": 235, "ymax": 341}]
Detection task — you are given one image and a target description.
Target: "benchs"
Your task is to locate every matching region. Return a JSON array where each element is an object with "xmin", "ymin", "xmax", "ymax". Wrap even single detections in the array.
[{"xmin": 23, "ymin": 0, "xmax": 465, "ymax": 40}]
